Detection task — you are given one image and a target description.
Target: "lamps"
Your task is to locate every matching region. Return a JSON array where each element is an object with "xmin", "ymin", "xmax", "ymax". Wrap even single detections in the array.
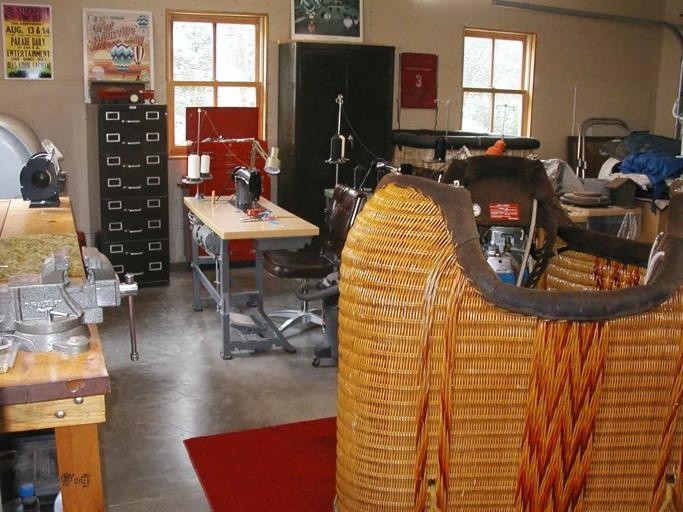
[{"xmin": 250, "ymin": 140, "xmax": 282, "ymax": 176}]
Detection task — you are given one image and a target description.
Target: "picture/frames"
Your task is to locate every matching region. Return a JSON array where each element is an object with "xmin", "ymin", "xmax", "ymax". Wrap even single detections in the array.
[
  {"xmin": 566, "ymin": 135, "xmax": 624, "ymax": 178},
  {"xmin": 289, "ymin": 0, "xmax": 364, "ymax": 43},
  {"xmin": 81, "ymin": 7, "xmax": 155, "ymax": 105},
  {"xmin": 0, "ymin": 2, "xmax": 55, "ymax": 81}
]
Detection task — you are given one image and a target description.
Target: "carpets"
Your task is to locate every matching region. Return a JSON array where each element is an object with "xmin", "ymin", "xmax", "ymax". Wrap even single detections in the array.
[{"xmin": 183, "ymin": 415, "xmax": 337, "ymax": 511}]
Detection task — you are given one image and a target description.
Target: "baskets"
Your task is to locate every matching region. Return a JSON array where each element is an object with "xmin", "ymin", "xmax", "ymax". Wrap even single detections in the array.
[{"xmin": 335, "ymin": 155, "xmax": 683, "ymax": 512}]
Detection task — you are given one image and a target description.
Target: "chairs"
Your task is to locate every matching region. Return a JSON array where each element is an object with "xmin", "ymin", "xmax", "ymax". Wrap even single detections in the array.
[{"xmin": 262, "ymin": 183, "xmax": 368, "ymax": 337}]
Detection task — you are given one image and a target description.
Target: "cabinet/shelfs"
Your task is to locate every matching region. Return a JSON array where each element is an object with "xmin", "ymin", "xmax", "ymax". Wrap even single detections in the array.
[
  {"xmin": 97, "ymin": 103, "xmax": 171, "ymax": 288},
  {"xmin": 276, "ymin": 39, "xmax": 396, "ymax": 227},
  {"xmin": 184, "ymin": 105, "xmax": 271, "ymax": 262}
]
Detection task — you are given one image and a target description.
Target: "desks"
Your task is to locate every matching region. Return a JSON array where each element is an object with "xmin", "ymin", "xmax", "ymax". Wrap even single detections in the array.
[
  {"xmin": 183, "ymin": 194, "xmax": 320, "ymax": 359},
  {"xmin": 1, "ymin": 196, "xmax": 113, "ymax": 512}
]
